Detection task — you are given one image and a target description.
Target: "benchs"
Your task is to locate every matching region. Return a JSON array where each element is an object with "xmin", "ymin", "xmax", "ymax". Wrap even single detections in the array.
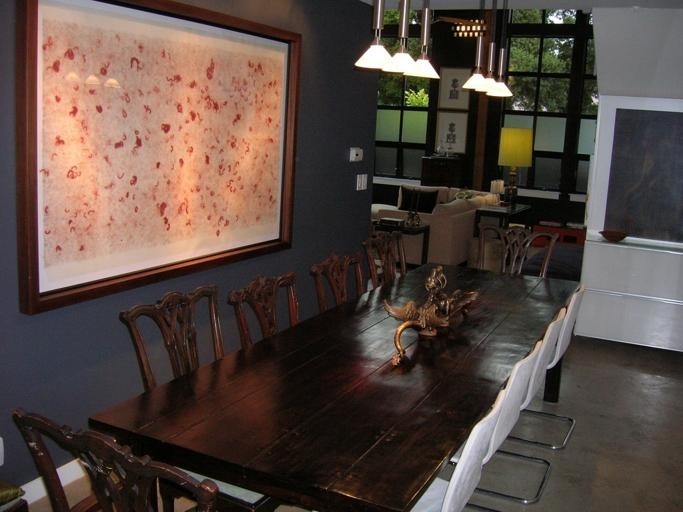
[{"xmin": 516, "ymin": 241, "xmax": 583, "ymax": 281}]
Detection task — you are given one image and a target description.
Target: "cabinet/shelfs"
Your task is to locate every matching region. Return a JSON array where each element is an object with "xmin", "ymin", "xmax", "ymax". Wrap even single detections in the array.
[
  {"xmin": 421, "ymin": 152, "xmax": 473, "ymax": 189},
  {"xmin": 571, "ymin": 234, "xmax": 683, "ymax": 359}
]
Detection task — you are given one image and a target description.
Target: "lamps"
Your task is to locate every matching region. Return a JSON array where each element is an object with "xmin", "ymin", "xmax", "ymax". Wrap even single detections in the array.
[
  {"xmin": 461, "ymin": 0, "xmax": 514, "ymax": 98},
  {"xmin": 352, "ymin": 0, "xmax": 440, "ymax": 80},
  {"xmin": 495, "ymin": 126, "xmax": 532, "ymax": 207}
]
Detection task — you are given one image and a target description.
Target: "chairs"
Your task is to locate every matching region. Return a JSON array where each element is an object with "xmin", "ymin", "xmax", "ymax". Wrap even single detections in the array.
[
  {"xmin": 412, "ymin": 285, "xmax": 583, "ymax": 512},
  {"xmin": 309, "ymin": 250, "xmax": 366, "ymax": 313},
  {"xmin": 226, "ymin": 271, "xmax": 301, "ymax": 351},
  {"xmin": 116, "ymin": 283, "xmax": 279, "ymax": 511},
  {"xmin": 360, "ymin": 231, "xmax": 407, "ymax": 287},
  {"xmin": 475, "ymin": 222, "xmax": 560, "ymax": 279},
  {"xmin": 12, "ymin": 406, "xmax": 217, "ymax": 512}
]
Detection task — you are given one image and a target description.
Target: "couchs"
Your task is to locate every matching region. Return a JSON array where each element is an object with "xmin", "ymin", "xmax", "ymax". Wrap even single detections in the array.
[{"xmin": 370, "ymin": 186, "xmax": 494, "ymax": 265}]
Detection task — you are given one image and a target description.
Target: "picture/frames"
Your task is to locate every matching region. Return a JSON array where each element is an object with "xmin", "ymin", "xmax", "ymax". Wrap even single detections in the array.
[{"xmin": 20, "ymin": 0, "xmax": 303, "ymax": 315}]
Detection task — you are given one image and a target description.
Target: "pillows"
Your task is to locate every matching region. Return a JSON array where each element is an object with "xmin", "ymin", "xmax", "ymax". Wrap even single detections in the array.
[
  {"xmin": 372, "ymin": 184, "xmax": 400, "ymax": 206},
  {"xmin": 398, "ymin": 187, "xmax": 438, "ymax": 214}
]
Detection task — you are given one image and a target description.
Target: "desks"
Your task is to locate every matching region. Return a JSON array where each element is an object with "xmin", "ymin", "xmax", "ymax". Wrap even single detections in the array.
[
  {"xmin": 373, "ymin": 219, "xmax": 430, "ymax": 264},
  {"xmin": 472, "ymin": 203, "xmax": 533, "ymax": 266}
]
